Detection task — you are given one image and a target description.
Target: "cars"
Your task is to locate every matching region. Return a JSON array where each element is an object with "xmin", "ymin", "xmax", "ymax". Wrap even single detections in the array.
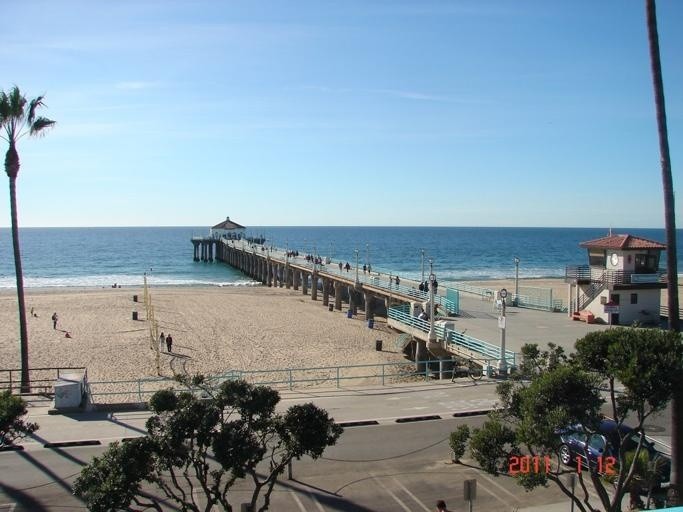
[{"xmin": 556, "ymin": 420, "xmax": 670, "ymax": 490}]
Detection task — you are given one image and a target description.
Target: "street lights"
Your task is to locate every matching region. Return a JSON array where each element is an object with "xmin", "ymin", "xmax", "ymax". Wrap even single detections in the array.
[{"xmin": 282, "ymin": 236, "xmax": 520, "ymax": 327}]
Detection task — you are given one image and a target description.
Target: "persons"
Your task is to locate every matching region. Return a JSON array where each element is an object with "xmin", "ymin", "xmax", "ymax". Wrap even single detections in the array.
[
  {"xmin": 286, "ymin": 248, "xmax": 438, "ymax": 294},
  {"xmin": 435, "ymin": 500, "xmax": 446, "ymax": 512},
  {"xmin": 159, "ymin": 332, "xmax": 166, "ymax": 353},
  {"xmin": 166, "ymin": 334, "xmax": 172, "ymax": 352},
  {"xmin": 31, "ymin": 306, "xmax": 34, "ymax": 315},
  {"xmin": 52, "ymin": 312, "xmax": 58, "ymax": 329}
]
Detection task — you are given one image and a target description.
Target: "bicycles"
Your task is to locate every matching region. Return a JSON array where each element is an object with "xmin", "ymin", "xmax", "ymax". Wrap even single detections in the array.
[{"xmin": 451, "ymin": 355, "xmax": 483, "ymax": 382}]
